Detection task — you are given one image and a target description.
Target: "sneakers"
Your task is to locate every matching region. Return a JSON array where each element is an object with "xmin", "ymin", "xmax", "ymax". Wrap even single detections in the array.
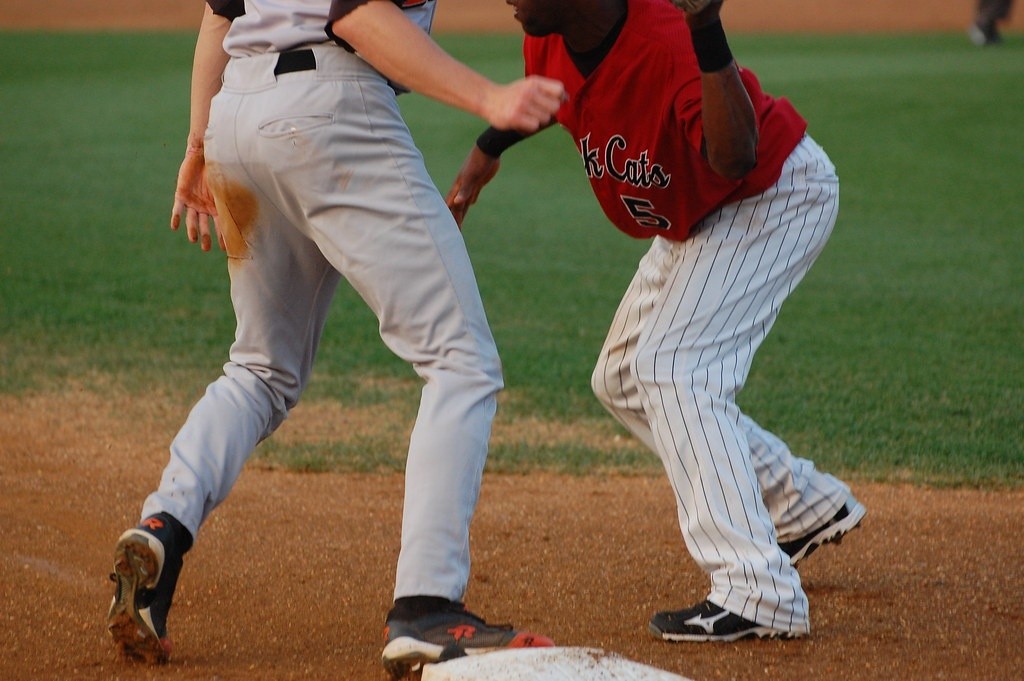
[
  {"xmin": 648, "ymin": 600, "xmax": 804, "ymax": 641},
  {"xmin": 381, "ymin": 602, "xmax": 557, "ymax": 679},
  {"xmin": 105, "ymin": 513, "xmax": 183, "ymax": 665},
  {"xmin": 775, "ymin": 489, "xmax": 868, "ymax": 565}
]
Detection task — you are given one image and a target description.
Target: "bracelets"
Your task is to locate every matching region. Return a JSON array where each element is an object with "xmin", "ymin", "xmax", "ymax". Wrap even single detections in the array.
[
  {"xmin": 688, "ymin": 15, "xmax": 733, "ymax": 73},
  {"xmin": 475, "ymin": 124, "xmax": 524, "ymax": 157}
]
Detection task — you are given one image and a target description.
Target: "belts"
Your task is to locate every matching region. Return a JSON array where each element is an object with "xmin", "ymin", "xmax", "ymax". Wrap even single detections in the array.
[{"xmin": 274, "ymin": 49, "xmax": 316, "ymax": 75}]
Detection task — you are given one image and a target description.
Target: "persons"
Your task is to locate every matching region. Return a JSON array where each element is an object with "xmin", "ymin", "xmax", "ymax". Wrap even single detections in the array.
[
  {"xmin": 968, "ymin": 0, "xmax": 1013, "ymax": 48},
  {"xmin": 441, "ymin": 1, "xmax": 866, "ymax": 642},
  {"xmin": 103, "ymin": 0, "xmax": 566, "ymax": 681}
]
